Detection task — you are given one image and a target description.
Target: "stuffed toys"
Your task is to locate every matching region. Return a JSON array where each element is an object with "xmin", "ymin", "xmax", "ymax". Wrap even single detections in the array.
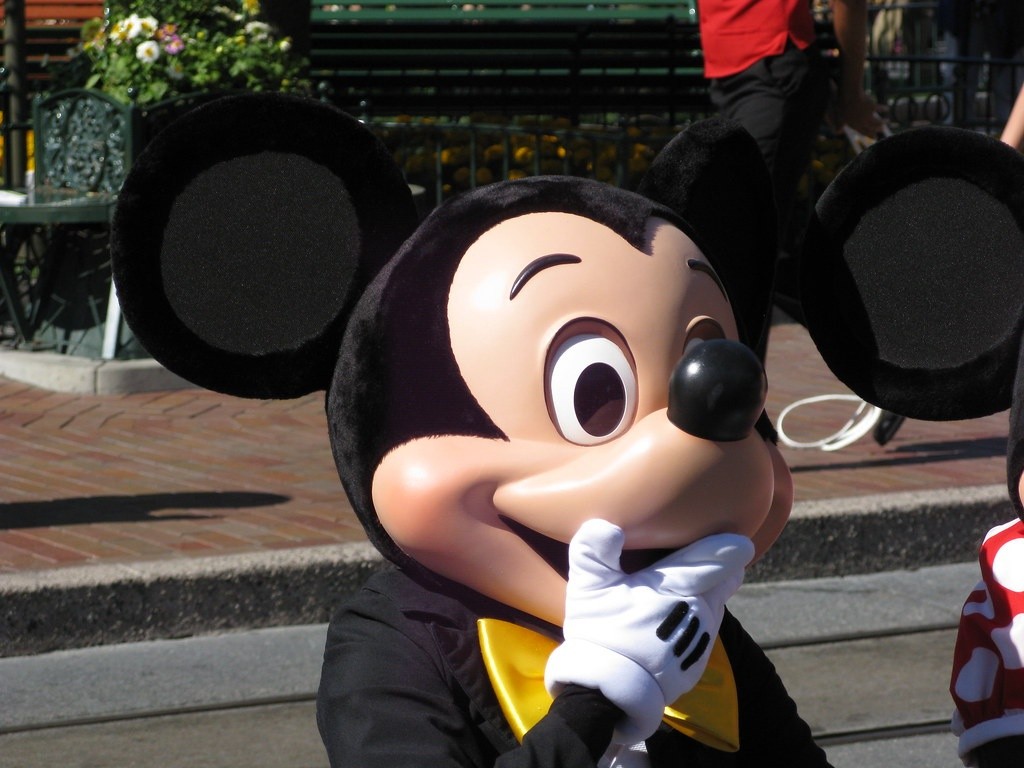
[{"xmin": 109, "ymin": 93, "xmax": 1024, "ymax": 768}]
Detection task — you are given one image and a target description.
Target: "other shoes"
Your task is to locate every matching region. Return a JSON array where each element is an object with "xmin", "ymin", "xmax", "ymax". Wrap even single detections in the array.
[{"xmin": 873, "ymin": 413, "xmax": 905, "ymax": 445}]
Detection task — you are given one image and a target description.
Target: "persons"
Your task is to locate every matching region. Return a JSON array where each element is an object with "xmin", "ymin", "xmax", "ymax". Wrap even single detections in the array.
[{"xmin": 696, "ymin": 0, "xmax": 908, "ymax": 448}]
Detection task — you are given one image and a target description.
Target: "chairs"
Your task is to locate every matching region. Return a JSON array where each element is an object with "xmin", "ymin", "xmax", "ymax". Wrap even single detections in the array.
[{"xmin": 0, "ymin": 76, "xmax": 138, "ymax": 357}]
